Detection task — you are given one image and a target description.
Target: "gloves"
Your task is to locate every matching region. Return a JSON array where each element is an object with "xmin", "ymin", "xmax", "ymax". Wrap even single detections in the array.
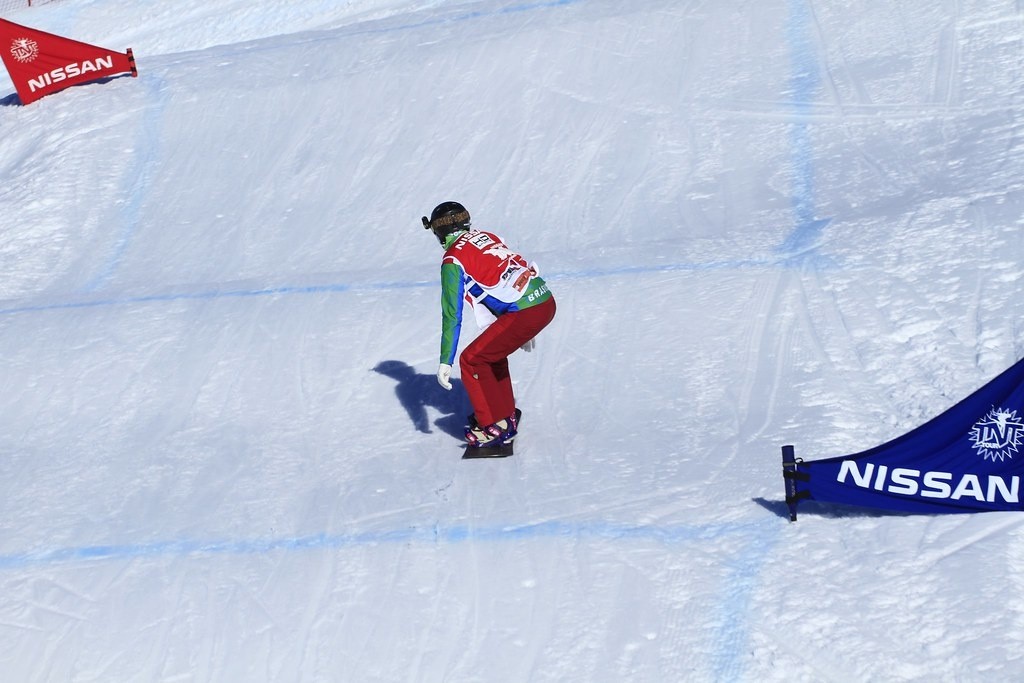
[
  {"xmin": 520, "ymin": 338, "xmax": 535, "ymax": 352},
  {"xmin": 437, "ymin": 363, "xmax": 453, "ymax": 390}
]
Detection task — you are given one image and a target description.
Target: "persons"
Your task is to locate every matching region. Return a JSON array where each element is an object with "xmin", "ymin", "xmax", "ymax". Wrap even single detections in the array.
[{"xmin": 422, "ymin": 201, "xmax": 556, "ymax": 456}]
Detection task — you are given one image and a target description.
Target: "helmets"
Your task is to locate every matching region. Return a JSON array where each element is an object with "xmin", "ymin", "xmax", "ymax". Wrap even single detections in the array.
[{"xmin": 430, "ymin": 201, "xmax": 470, "ymax": 246}]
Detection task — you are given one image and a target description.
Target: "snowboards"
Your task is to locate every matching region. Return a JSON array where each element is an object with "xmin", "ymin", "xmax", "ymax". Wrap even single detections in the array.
[{"xmin": 461, "ymin": 407, "xmax": 522, "ymax": 459}]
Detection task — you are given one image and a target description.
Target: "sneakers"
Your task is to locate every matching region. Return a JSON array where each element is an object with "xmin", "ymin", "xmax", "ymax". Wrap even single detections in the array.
[
  {"xmin": 467, "ymin": 412, "xmax": 517, "ymax": 431},
  {"xmin": 464, "ymin": 418, "xmax": 517, "ymax": 446}
]
